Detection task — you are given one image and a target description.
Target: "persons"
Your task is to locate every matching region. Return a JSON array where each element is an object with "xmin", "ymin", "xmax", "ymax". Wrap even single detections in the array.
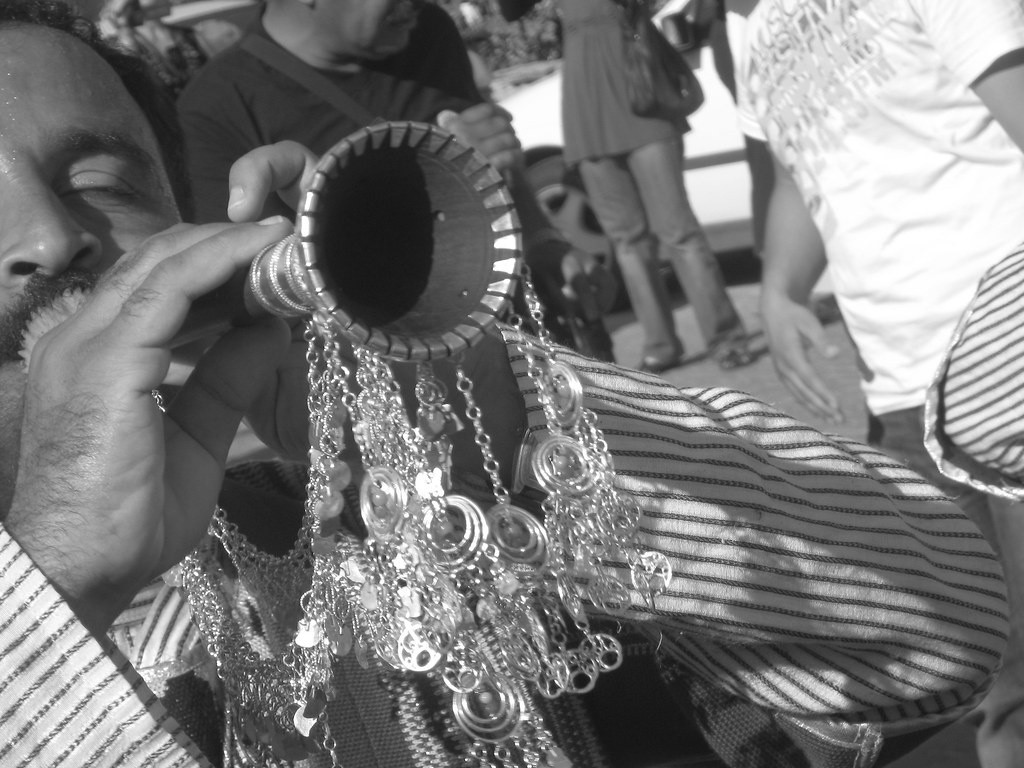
[
  {"xmin": 2, "ymin": 0, "xmax": 1005, "ymax": 767},
  {"xmin": 724, "ymin": 0, "xmax": 1024, "ymax": 540},
  {"xmin": 541, "ymin": 0, "xmax": 754, "ymax": 373},
  {"xmin": 177, "ymin": 1, "xmax": 620, "ymax": 368},
  {"xmin": 923, "ymin": 248, "xmax": 1023, "ymax": 499}
]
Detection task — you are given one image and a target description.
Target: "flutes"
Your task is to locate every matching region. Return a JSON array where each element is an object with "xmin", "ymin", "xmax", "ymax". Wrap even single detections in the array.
[{"xmin": 168, "ymin": 120, "xmax": 673, "ymax": 766}]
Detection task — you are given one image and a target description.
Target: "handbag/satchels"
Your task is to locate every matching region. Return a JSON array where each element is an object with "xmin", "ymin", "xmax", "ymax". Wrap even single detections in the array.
[{"xmin": 619, "ymin": 0, "xmax": 704, "ymax": 117}]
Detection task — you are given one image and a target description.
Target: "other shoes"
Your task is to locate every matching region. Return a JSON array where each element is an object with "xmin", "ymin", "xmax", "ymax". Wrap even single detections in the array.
[{"xmin": 638, "ymin": 345, "xmax": 749, "ymax": 375}]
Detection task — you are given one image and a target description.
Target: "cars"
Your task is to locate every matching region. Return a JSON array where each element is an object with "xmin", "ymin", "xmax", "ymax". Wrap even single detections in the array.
[{"xmin": 493, "ymin": 0, "xmax": 765, "ymax": 323}]
